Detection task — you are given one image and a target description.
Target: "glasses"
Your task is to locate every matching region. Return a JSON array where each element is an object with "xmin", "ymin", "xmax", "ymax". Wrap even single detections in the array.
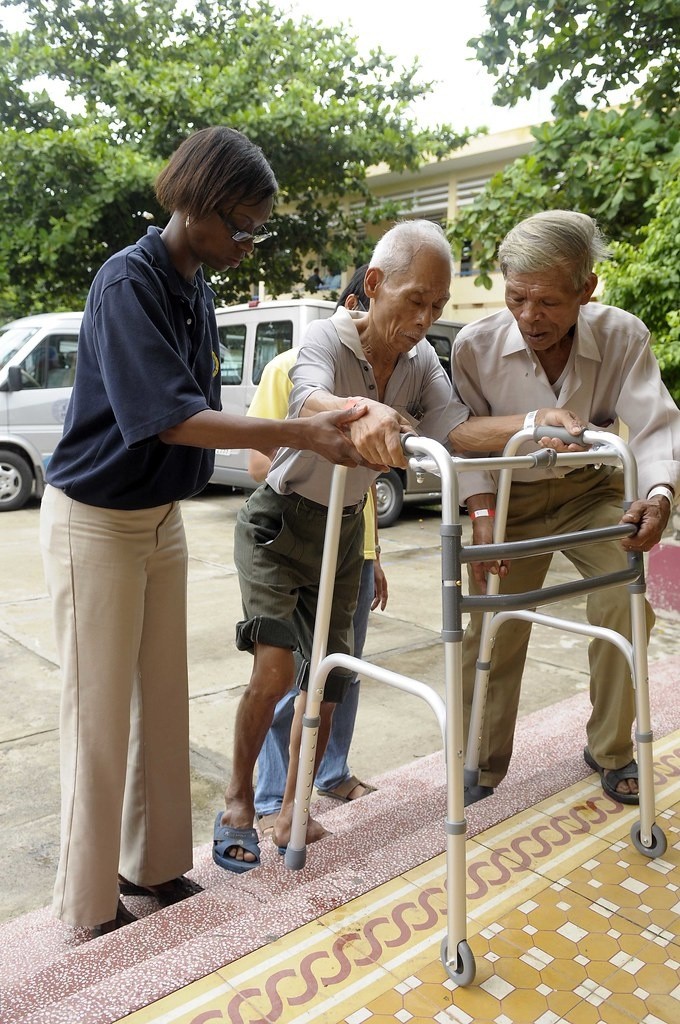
[
  {"xmin": 410, "ymin": 409, "xmax": 425, "ymax": 422},
  {"xmin": 213, "ymin": 205, "xmax": 272, "ymax": 244}
]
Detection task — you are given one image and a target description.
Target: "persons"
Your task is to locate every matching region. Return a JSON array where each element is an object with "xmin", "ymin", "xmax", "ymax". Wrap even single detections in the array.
[
  {"xmin": 212, "ymin": 211, "xmax": 680, "ymax": 873},
  {"xmin": 37, "ymin": 129, "xmax": 390, "ymax": 934}
]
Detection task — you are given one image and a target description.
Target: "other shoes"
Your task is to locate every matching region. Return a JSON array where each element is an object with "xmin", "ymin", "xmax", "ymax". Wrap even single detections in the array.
[
  {"xmin": 89, "ymin": 898, "xmax": 139, "ymax": 939},
  {"xmin": 118, "ymin": 873, "xmax": 206, "ymax": 908}
]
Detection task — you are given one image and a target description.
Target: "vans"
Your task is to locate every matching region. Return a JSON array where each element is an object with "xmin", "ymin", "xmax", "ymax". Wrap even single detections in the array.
[
  {"xmin": 0, "ymin": 311, "xmax": 84, "ymax": 511},
  {"xmin": 207, "ymin": 293, "xmax": 468, "ymax": 531}
]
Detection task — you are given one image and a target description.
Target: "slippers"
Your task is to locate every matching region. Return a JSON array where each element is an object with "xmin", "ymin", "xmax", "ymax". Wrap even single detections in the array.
[
  {"xmin": 212, "ymin": 812, "xmax": 263, "ymax": 874},
  {"xmin": 583, "ymin": 745, "xmax": 640, "ymax": 806},
  {"xmin": 317, "ymin": 775, "xmax": 378, "ymax": 804},
  {"xmin": 465, "ymin": 785, "xmax": 495, "ymax": 808},
  {"xmin": 256, "ymin": 811, "xmax": 280, "ymax": 837},
  {"xmin": 277, "ymin": 832, "xmax": 335, "ymax": 856}
]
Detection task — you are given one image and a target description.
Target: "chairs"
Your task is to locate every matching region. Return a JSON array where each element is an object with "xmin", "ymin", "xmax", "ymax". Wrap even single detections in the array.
[{"xmin": 35, "ymin": 345, "xmax": 78, "ymax": 387}]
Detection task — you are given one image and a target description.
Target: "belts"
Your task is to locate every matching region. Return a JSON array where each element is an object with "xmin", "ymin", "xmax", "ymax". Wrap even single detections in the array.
[
  {"xmin": 283, "ymin": 491, "xmax": 369, "ymax": 516},
  {"xmin": 557, "ymin": 464, "xmax": 606, "ymax": 480}
]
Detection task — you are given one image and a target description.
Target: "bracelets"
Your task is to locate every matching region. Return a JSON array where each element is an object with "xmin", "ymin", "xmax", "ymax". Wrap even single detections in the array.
[
  {"xmin": 471, "ymin": 509, "xmax": 495, "ymax": 523},
  {"xmin": 342, "ymin": 395, "xmax": 364, "ymax": 410},
  {"xmin": 524, "ymin": 409, "xmax": 539, "ymax": 430},
  {"xmin": 376, "ymin": 545, "xmax": 381, "ymax": 553},
  {"xmin": 647, "ymin": 487, "xmax": 675, "ymax": 516}
]
